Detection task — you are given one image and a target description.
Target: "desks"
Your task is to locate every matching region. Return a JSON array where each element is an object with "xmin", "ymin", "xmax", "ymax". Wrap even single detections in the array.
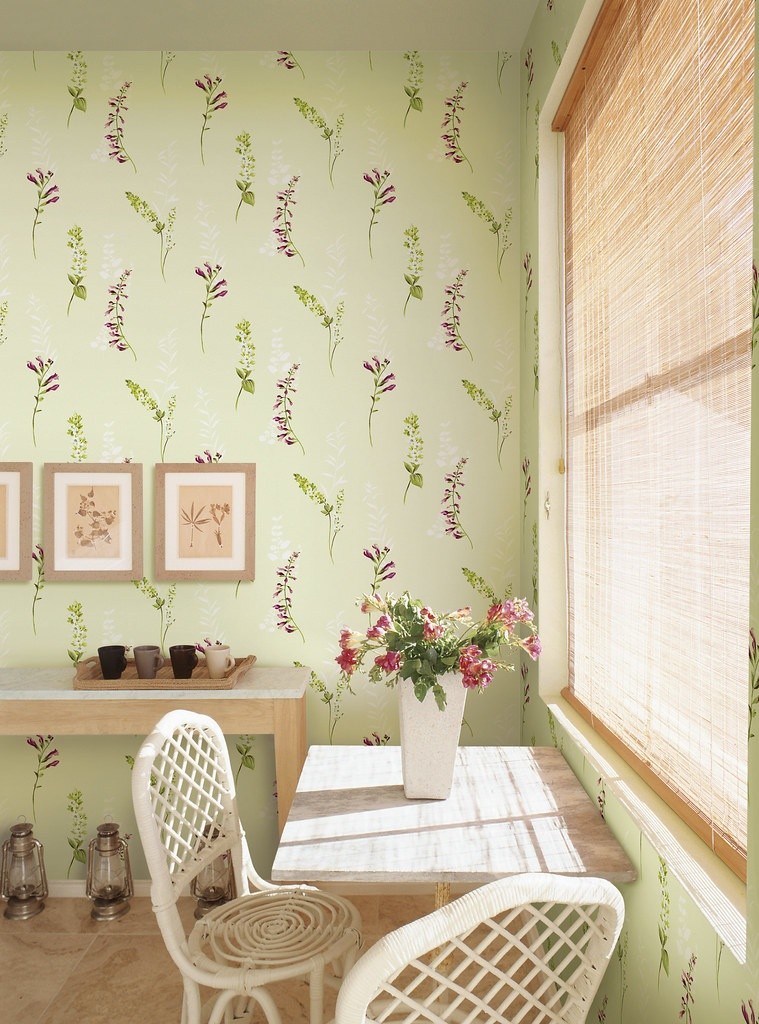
[
  {"xmin": 270, "ymin": 744, "xmax": 640, "ymax": 1013},
  {"xmin": 0, "ymin": 666, "xmax": 314, "ymax": 837}
]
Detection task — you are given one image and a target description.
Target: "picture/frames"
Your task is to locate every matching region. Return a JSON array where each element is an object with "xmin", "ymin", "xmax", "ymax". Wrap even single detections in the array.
[
  {"xmin": 154, "ymin": 463, "xmax": 256, "ymax": 582},
  {"xmin": 43, "ymin": 462, "xmax": 144, "ymax": 582},
  {"xmin": 0, "ymin": 461, "xmax": 34, "ymax": 583}
]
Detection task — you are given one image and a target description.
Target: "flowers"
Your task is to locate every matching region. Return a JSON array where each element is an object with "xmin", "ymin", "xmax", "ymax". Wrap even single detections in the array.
[{"xmin": 335, "ymin": 567, "xmax": 544, "ymax": 710}]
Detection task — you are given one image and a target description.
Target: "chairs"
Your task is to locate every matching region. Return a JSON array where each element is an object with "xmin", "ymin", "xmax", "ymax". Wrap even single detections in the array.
[
  {"xmin": 131, "ymin": 709, "xmax": 363, "ymax": 1024},
  {"xmin": 325, "ymin": 873, "xmax": 626, "ymax": 1024}
]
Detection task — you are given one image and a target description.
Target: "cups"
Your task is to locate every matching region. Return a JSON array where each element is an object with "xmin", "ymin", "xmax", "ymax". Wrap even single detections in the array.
[
  {"xmin": 134, "ymin": 645, "xmax": 165, "ymax": 679},
  {"xmin": 97, "ymin": 646, "xmax": 127, "ymax": 679},
  {"xmin": 206, "ymin": 645, "xmax": 235, "ymax": 679},
  {"xmin": 169, "ymin": 645, "xmax": 198, "ymax": 679}
]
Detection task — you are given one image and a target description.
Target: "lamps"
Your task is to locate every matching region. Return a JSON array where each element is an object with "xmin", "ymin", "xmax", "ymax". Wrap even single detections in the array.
[{"xmin": 0, "ymin": 815, "xmax": 237, "ymax": 924}]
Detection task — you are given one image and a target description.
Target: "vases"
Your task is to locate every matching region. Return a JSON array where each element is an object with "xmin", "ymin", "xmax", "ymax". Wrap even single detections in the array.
[{"xmin": 396, "ymin": 669, "xmax": 471, "ymax": 802}]
